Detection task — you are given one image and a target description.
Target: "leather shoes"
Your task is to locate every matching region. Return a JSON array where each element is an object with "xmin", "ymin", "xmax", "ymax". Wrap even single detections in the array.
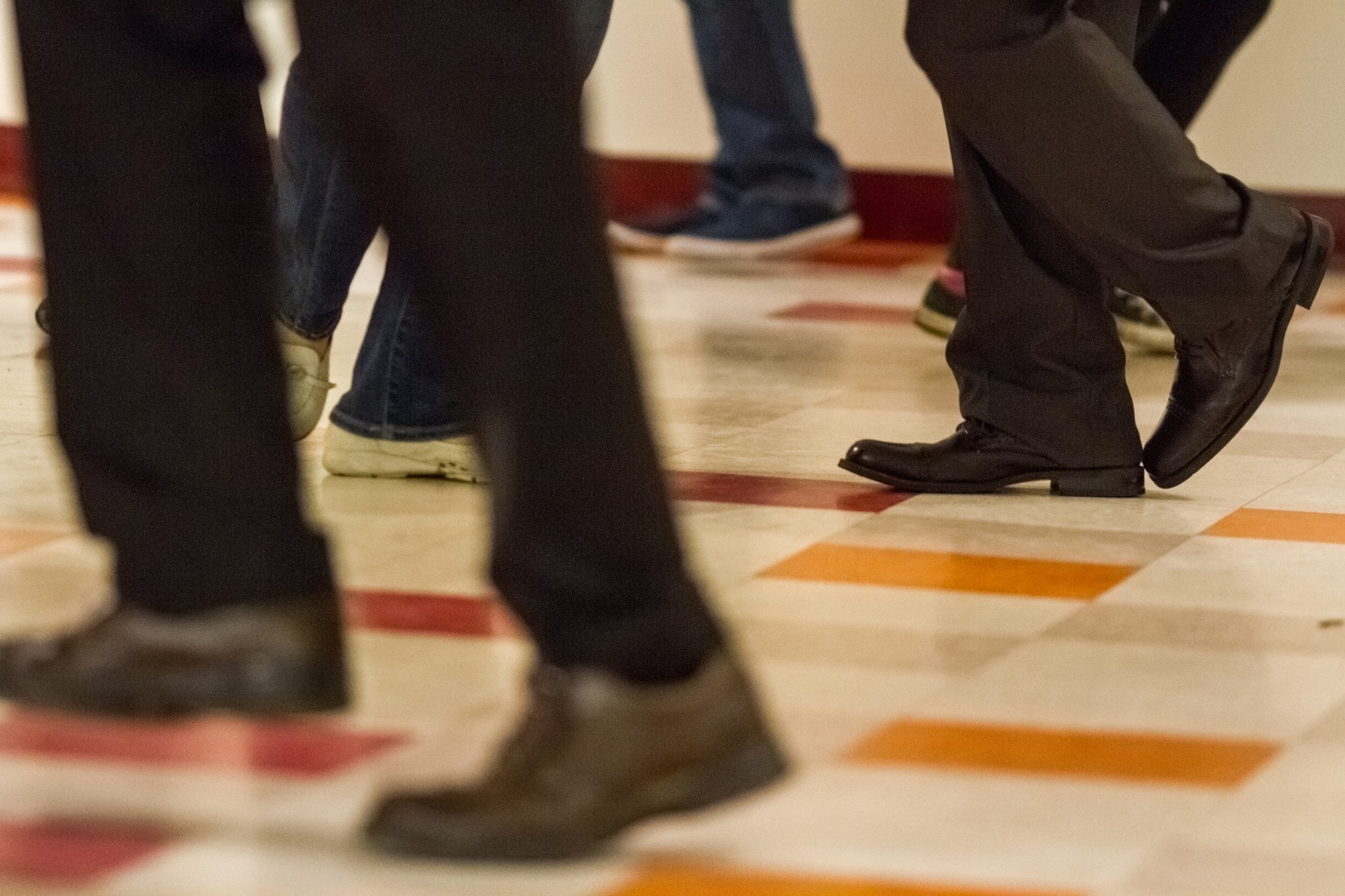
[
  {"xmin": 837, "ymin": 417, "xmax": 1144, "ymax": 498},
  {"xmin": 1147, "ymin": 212, "xmax": 1333, "ymax": 489}
]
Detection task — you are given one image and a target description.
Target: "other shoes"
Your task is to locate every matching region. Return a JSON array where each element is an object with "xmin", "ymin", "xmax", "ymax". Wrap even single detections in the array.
[
  {"xmin": 365, "ymin": 652, "xmax": 788, "ymax": 856},
  {"xmin": 323, "ymin": 419, "xmax": 489, "ymax": 485},
  {"xmin": 274, "ymin": 321, "xmax": 328, "ymax": 439},
  {"xmin": 0, "ymin": 568, "xmax": 345, "ymax": 718},
  {"xmin": 667, "ymin": 196, "xmax": 861, "ymax": 259},
  {"xmin": 607, "ymin": 204, "xmax": 705, "ymax": 252}
]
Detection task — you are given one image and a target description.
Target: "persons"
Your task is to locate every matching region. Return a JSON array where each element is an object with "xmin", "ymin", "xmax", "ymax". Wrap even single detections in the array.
[
  {"xmin": 0, "ymin": 0, "xmax": 788, "ymax": 866},
  {"xmin": 597, "ymin": 0, "xmax": 862, "ymax": 261},
  {"xmin": 913, "ymin": 0, "xmax": 1277, "ymax": 354},
  {"xmin": 832, "ymin": 0, "xmax": 1335, "ymax": 496},
  {"xmin": 243, "ymin": 0, "xmax": 616, "ymax": 490}
]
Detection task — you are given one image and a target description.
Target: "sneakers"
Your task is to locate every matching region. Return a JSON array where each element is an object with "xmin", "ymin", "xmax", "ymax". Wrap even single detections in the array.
[
  {"xmin": 915, "ymin": 268, "xmax": 968, "ymax": 338},
  {"xmin": 1110, "ymin": 288, "xmax": 1176, "ymax": 353}
]
